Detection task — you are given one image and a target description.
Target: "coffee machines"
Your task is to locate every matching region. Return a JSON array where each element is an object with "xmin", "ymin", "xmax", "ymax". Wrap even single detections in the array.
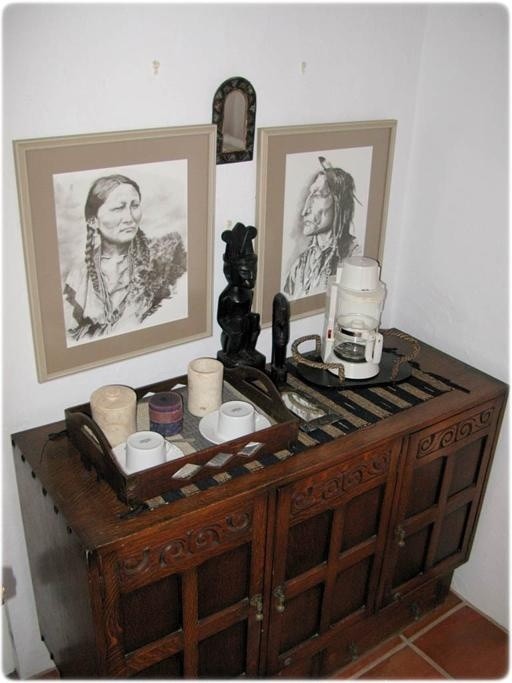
[{"xmin": 320, "ymin": 256, "xmax": 385, "ymax": 379}]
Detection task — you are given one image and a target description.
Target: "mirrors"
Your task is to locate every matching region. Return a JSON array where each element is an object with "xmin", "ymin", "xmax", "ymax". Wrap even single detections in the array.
[{"xmin": 213, "ymin": 75, "xmax": 258, "ymax": 166}]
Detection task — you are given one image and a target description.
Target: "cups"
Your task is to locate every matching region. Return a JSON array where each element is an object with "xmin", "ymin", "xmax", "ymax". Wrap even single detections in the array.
[
  {"xmin": 89, "ymin": 384, "xmax": 138, "ymax": 446},
  {"xmin": 186, "ymin": 357, "xmax": 225, "ymax": 417},
  {"xmin": 216, "ymin": 399, "xmax": 256, "ymax": 440},
  {"xmin": 147, "ymin": 391, "xmax": 184, "ymax": 437},
  {"xmin": 125, "ymin": 431, "xmax": 170, "ymax": 473}
]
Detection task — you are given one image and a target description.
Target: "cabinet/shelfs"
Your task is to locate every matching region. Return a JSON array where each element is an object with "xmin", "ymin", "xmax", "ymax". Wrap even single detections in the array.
[{"xmin": 11, "ymin": 323, "xmax": 508, "ymax": 678}]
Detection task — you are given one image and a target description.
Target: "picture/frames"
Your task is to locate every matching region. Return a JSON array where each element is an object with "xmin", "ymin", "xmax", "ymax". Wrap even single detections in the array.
[
  {"xmin": 248, "ymin": 121, "xmax": 397, "ymax": 329},
  {"xmin": 12, "ymin": 123, "xmax": 223, "ymax": 385}
]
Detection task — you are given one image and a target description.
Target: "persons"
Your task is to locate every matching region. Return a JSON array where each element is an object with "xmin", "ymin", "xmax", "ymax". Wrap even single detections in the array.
[
  {"xmin": 61, "ymin": 174, "xmax": 188, "ymax": 343},
  {"xmin": 218, "ymin": 222, "xmax": 261, "ymax": 355},
  {"xmin": 283, "ymin": 155, "xmax": 362, "ymax": 296}
]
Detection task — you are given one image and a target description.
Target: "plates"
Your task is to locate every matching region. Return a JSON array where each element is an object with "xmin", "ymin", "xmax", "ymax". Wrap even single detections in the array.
[
  {"xmin": 112, "ymin": 439, "xmax": 184, "ymax": 475},
  {"xmin": 198, "ymin": 410, "xmax": 271, "ymax": 445}
]
